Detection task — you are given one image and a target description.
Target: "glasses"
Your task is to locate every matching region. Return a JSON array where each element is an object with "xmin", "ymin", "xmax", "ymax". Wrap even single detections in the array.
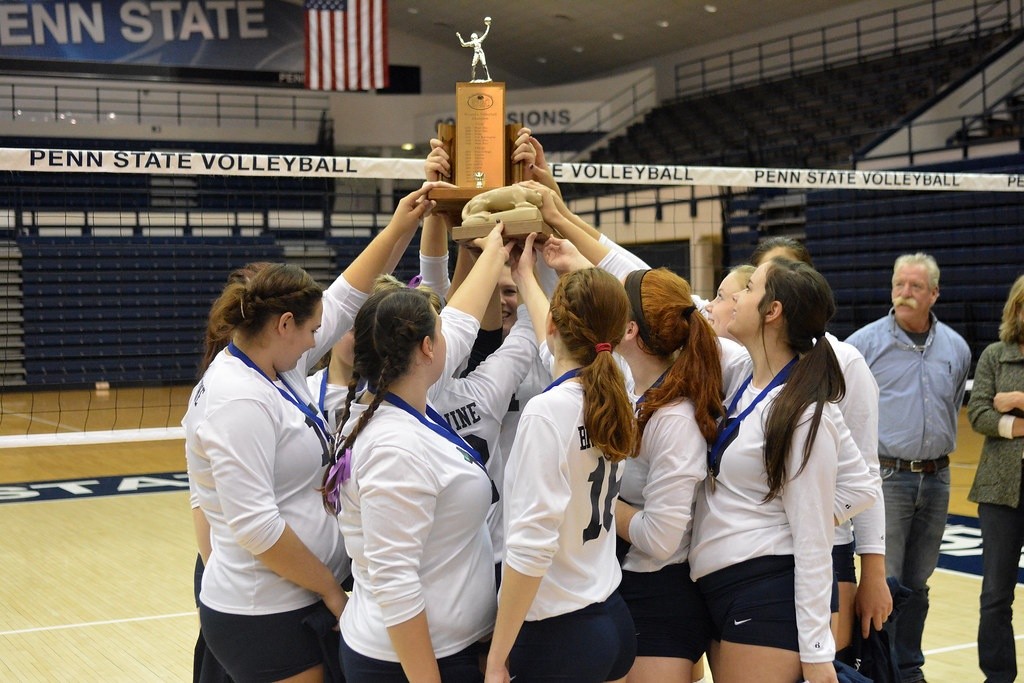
[{"xmin": 887, "ymin": 318, "xmax": 933, "ymax": 352}]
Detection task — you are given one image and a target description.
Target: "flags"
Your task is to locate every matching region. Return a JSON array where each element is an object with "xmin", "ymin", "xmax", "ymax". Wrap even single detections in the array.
[{"xmin": 303, "ymin": 0, "xmax": 389, "ymax": 92}]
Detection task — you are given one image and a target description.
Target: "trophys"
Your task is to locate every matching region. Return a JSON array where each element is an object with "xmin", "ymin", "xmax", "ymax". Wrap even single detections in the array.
[{"xmin": 428, "ymin": 17, "xmax": 525, "ymax": 212}]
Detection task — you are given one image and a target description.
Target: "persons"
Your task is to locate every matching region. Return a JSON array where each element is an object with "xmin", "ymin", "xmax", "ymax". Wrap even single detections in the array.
[
  {"xmin": 844, "ymin": 252, "xmax": 973, "ymax": 683},
  {"xmin": 180, "ymin": 128, "xmax": 893, "ymax": 683},
  {"xmin": 966, "ymin": 274, "xmax": 1024, "ymax": 683}
]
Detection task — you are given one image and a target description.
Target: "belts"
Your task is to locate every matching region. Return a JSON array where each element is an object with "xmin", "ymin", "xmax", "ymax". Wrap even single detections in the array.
[{"xmin": 879, "ymin": 457, "xmax": 949, "ymax": 472}]
